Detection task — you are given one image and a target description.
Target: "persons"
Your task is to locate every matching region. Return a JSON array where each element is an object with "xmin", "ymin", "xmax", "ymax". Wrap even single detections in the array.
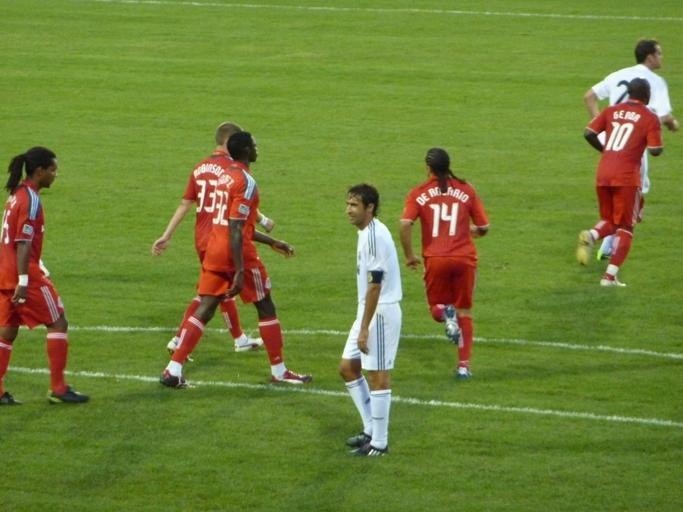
[
  {"xmin": 151, "ymin": 123, "xmax": 275, "ymax": 362},
  {"xmin": 576, "ymin": 78, "xmax": 664, "ymax": 287},
  {"xmin": 583, "ymin": 40, "xmax": 679, "ymax": 260},
  {"xmin": 0, "ymin": 148, "xmax": 89, "ymax": 405},
  {"xmin": 399, "ymin": 148, "xmax": 490, "ymax": 382},
  {"xmin": 338, "ymin": 185, "xmax": 402, "ymax": 458},
  {"xmin": 159, "ymin": 132, "xmax": 313, "ymax": 389}
]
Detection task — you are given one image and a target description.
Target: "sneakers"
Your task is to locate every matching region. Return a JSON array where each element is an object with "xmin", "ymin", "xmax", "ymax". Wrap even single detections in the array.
[
  {"xmin": 455, "ymin": 369, "xmax": 472, "ymax": 380},
  {"xmin": 576, "ymin": 230, "xmax": 591, "ymax": 264},
  {"xmin": 444, "ymin": 304, "xmax": 462, "ymax": 344},
  {"xmin": 0, "ymin": 391, "xmax": 22, "ymax": 405},
  {"xmin": 167, "ymin": 336, "xmax": 193, "ymax": 362},
  {"xmin": 271, "ymin": 369, "xmax": 312, "ymax": 385},
  {"xmin": 596, "ymin": 248, "xmax": 612, "ymax": 261},
  {"xmin": 346, "ymin": 432, "xmax": 371, "ymax": 447},
  {"xmin": 600, "ymin": 273, "xmax": 626, "ymax": 286},
  {"xmin": 235, "ymin": 337, "xmax": 263, "ymax": 352},
  {"xmin": 348, "ymin": 442, "xmax": 389, "ymax": 457},
  {"xmin": 160, "ymin": 369, "xmax": 188, "ymax": 389},
  {"xmin": 48, "ymin": 384, "xmax": 89, "ymax": 403}
]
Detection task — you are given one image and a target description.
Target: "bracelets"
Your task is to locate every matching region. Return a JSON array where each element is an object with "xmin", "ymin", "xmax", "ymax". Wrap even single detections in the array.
[
  {"xmin": 259, "ymin": 216, "xmax": 268, "ymax": 226},
  {"xmin": 18, "ymin": 274, "xmax": 28, "ymax": 286}
]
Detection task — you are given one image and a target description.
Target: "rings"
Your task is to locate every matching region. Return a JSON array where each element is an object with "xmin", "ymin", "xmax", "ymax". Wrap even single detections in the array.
[{"xmin": 19, "ymin": 298, "xmax": 26, "ymax": 304}]
